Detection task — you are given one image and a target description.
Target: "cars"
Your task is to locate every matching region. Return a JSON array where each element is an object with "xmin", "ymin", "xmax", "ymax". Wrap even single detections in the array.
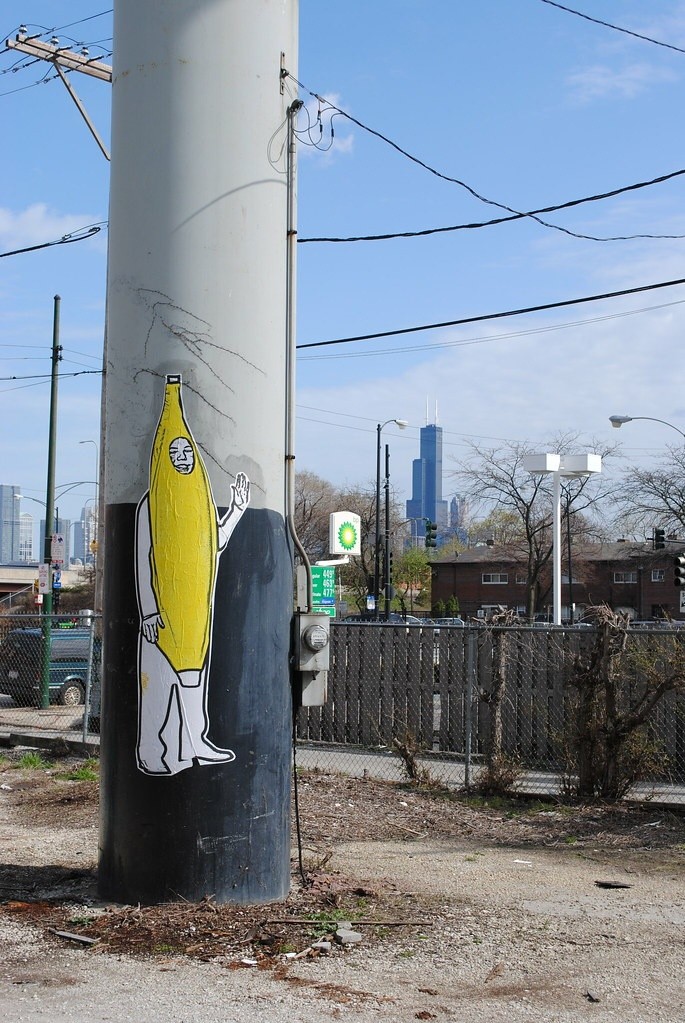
[{"xmin": 334, "ymin": 610, "xmax": 466, "ymax": 638}]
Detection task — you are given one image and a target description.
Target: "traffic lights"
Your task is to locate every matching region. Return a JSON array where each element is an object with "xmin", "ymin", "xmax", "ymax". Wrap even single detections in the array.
[
  {"xmin": 381, "ymin": 550, "xmax": 393, "ymax": 590},
  {"xmin": 673, "ymin": 555, "xmax": 685, "ymax": 587},
  {"xmin": 425, "ymin": 520, "xmax": 438, "ymax": 548},
  {"xmin": 54, "ymin": 589, "xmax": 61, "ymax": 607},
  {"xmin": 652, "ymin": 526, "xmax": 666, "ymax": 551}
]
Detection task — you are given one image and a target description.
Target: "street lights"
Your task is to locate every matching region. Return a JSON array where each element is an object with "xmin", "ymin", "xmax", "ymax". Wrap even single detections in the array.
[
  {"xmin": 522, "ymin": 452, "xmax": 603, "ymax": 626},
  {"xmin": 372, "ymin": 417, "xmax": 411, "ymax": 622},
  {"xmin": 520, "ymin": 484, "xmax": 575, "ymax": 625},
  {"xmin": 12, "ymin": 493, "xmax": 59, "ymax": 614},
  {"xmin": 78, "ymin": 439, "xmax": 99, "ymax": 571}
]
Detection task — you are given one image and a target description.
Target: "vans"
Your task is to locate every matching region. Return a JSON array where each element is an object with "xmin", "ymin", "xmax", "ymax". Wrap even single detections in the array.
[{"xmin": 0, "ymin": 623, "xmax": 95, "ymax": 708}]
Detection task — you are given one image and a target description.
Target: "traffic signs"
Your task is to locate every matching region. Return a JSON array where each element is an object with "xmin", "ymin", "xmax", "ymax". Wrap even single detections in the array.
[{"xmin": 679, "ymin": 590, "xmax": 685, "ymax": 614}]
